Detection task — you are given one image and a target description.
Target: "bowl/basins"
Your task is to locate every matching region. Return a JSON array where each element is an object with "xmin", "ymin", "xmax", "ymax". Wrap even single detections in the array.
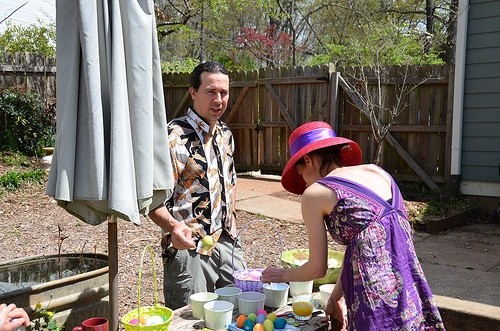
[{"xmin": 282, "ymin": 248, "xmax": 345, "ymax": 288}]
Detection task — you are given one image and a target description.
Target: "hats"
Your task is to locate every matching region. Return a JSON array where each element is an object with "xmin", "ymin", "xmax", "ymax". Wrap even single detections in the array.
[{"xmin": 281, "ymin": 122, "xmax": 362, "ymax": 194}]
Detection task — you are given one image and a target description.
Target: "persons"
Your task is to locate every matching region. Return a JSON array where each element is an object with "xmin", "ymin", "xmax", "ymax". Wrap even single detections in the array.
[
  {"xmin": 0, "ymin": 303, "xmax": 30, "ymax": 331},
  {"xmin": 148, "ymin": 61, "xmax": 247, "ymax": 311},
  {"xmin": 261, "ymin": 121, "xmax": 445, "ymax": 331}
]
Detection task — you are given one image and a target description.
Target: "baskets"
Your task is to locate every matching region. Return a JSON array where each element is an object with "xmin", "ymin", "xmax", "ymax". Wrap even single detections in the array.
[
  {"xmin": 120, "ymin": 245, "xmax": 174, "ymax": 330},
  {"xmin": 232, "ymin": 219, "xmax": 285, "ymax": 291}
]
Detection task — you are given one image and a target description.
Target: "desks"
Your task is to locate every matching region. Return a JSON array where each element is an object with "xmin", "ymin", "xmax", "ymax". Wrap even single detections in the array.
[{"xmin": 165, "ymin": 288, "xmax": 348, "ymax": 331}]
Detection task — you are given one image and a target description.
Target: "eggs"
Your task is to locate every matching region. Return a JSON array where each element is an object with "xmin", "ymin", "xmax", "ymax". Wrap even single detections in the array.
[
  {"xmin": 202, "ymin": 235, "xmax": 213, "ymax": 250},
  {"xmin": 129, "ymin": 314, "xmax": 164, "ymax": 325},
  {"xmin": 236, "ymin": 309, "xmax": 286, "ymax": 331}
]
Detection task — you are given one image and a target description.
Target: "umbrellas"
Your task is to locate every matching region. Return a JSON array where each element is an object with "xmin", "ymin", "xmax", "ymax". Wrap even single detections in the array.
[{"xmin": 44, "ymin": 0, "xmax": 175, "ymax": 331}]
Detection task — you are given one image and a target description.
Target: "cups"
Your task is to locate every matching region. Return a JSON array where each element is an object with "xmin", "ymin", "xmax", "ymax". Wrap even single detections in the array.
[
  {"xmin": 319, "ymin": 284, "xmax": 345, "ymax": 309},
  {"xmin": 291, "ymin": 292, "xmax": 314, "ymax": 320},
  {"xmin": 238, "ymin": 291, "xmax": 266, "ymax": 316},
  {"xmin": 72, "ymin": 317, "xmax": 109, "ymax": 331},
  {"xmin": 190, "ymin": 292, "xmax": 218, "ymax": 319},
  {"xmin": 262, "ymin": 282, "xmax": 290, "ymax": 308},
  {"xmin": 289, "ymin": 280, "xmax": 313, "ymax": 297},
  {"xmin": 214, "ymin": 287, "xmax": 242, "ymax": 310},
  {"xmin": 204, "ymin": 300, "xmax": 234, "ymax": 330}
]
